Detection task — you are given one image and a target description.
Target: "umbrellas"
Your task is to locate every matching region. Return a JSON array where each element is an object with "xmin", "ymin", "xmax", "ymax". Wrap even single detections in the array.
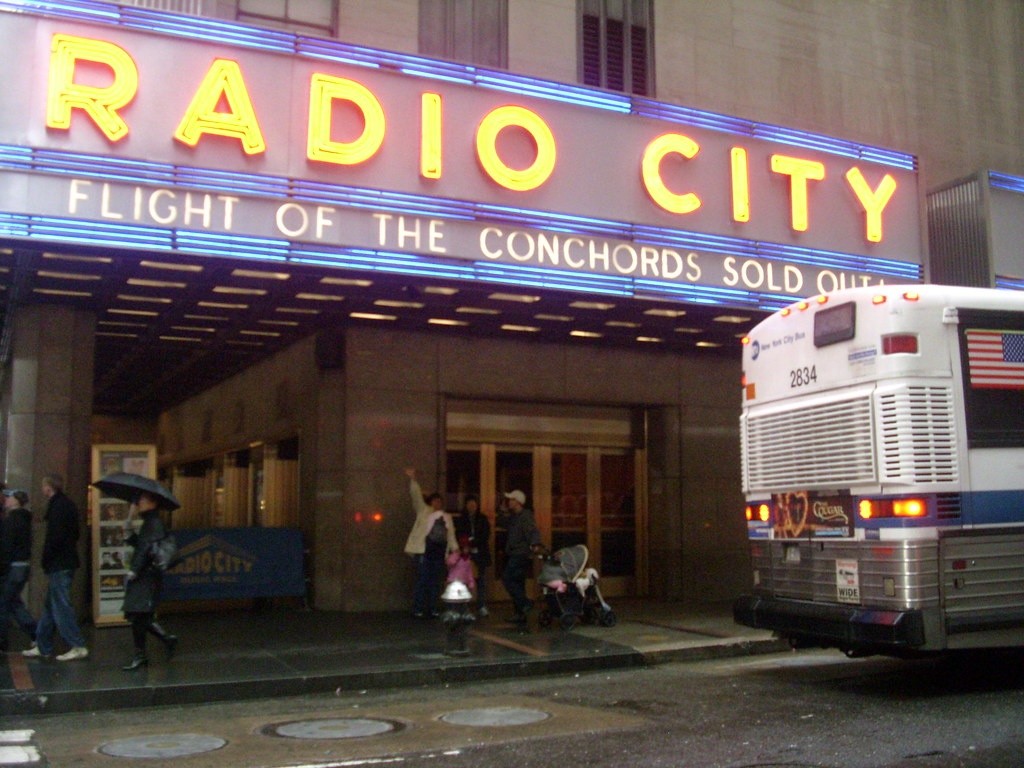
[{"xmin": 91, "ymin": 471, "xmax": 181, "ymax": 521}]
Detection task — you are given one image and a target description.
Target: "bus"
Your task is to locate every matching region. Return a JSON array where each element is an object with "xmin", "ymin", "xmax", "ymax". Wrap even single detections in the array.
[{"xmin": 732, "ymin": 283, "xmax": 1024, "ymax": 665}]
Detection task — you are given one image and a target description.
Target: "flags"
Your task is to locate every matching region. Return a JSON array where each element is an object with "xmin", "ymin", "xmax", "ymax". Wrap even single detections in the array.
[{"xmin": 967, "ymin": 329, "xmax": 1024, "ymax": 390}]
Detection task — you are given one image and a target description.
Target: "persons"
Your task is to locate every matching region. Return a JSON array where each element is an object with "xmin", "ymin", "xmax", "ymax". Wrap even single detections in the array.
[
  {"xmin": 20, "ymin": 473, "xmax": 89, "ymax": 662},
  {"xmin": 0, "ymin": 489, "xmax": 37, "ymax": 649},
  {"xmin": 122, "ymin": 489, "xmax": 178, "ymax": 672},
  {"xmin": 454, "ymin": 494, "xmax": 490, "ymax": 622},
  {"xmin": 402, "ymin": 466, "xmax": 460, "ymax": 619},
  {"xmin": 500, "ymin": 489, "xmax": 541, "ymax": 624},
  {"xmin": 445, "ymin": 533, "xmax": 477, "ymax": 620}
]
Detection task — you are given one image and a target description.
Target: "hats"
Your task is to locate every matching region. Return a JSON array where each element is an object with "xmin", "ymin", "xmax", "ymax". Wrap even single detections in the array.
[
  {"xmin": 504, "ymin": 490, "xmax": 525, "ymax": 505},
  {"xmin": 3, "ymin": 489, "xmax": 28, "ymax": 505},
  {"xmin": 458, "ymin": 532, "xmax": 470, "ymax": 547}
]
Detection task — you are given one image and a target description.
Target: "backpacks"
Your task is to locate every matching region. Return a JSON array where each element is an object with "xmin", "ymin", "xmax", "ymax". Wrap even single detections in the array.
[{"xmin": 428, "ymin": 515, "xmax": 448, "ymax": 547}]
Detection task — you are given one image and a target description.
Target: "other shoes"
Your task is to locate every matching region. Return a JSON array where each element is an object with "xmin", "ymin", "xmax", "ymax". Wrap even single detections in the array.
[
  {"xmin": 479, "ymin": 607, "xmax": 489, "ymax": 617},
  {"xmin": 165, "ymin": 634, "xmax": 176, "ymax": 661},
  {"xmin": 123, "ymin": 648, "xmax": 148, "ymax": 670},
  {"xmin": 507, "ymin": 612, "xmax": 528, "ymax": 623},
  {"xmin": 55, "ymin": 648, "xmax": 88, "ymax": 660},
  {"xmin": 22, "ymin": 647, "xmax": 49, "ymax": 658}
]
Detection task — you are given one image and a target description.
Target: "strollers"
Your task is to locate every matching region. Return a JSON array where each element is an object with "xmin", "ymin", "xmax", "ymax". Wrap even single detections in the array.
[{"xmin": 529, "ymin": 541, "xmax": 618, "ymax": 629}]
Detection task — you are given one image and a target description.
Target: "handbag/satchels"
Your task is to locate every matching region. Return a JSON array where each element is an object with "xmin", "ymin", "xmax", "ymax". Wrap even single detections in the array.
[{"xmin": 149, "ymin": 534, "xmax": 178, "ymax": 573}]
